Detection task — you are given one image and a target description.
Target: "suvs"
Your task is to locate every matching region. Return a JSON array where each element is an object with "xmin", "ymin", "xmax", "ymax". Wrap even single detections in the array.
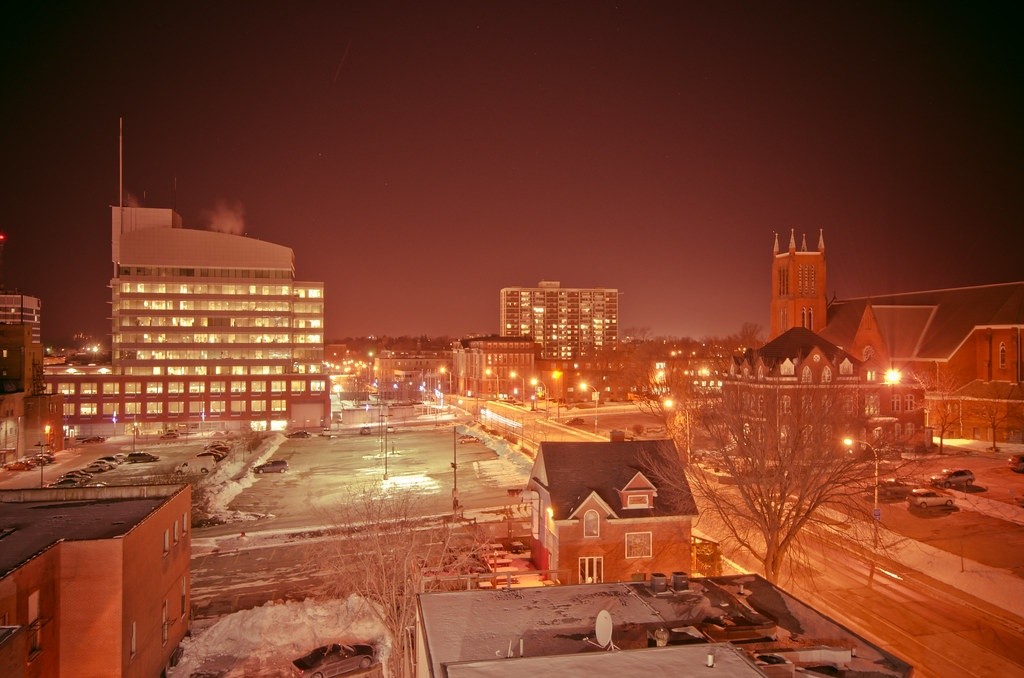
[
  {"xmin": 931, "ymin": 468, "xmax": 976, "ymax": 489},
  {"xmin": 905, "ymin": 488, "xmax": 956, "ymax": 509}
]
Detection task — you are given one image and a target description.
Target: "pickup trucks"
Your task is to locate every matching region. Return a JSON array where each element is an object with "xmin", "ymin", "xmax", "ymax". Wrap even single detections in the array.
[
  {"xmin": 254, "ymin": 460, "xmax": 289, "ymax": 474},
  {"xmin": 322, "ymin": 428, "xmax": 331, "ymax": 437}
]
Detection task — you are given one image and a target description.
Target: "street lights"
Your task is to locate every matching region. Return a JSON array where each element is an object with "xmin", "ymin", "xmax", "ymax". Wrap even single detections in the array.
[
  {"xmin": 379, "ymin": 413, "xmax": 393, "ymax": 480},
  {"xmin": 441, "ymin": 366, "xmax": 452, "ymax": 414},
  {"xmin": 845, "ymin": 440, "xmax": 879, "ymax": 589},
  {"xmin": 510, "ymin": 372, "xmax": 525, "ymax": 405},
  {"xmin": 530, "ymin": 379, "xmax": 549, "ymax": 421},
  {"xmin": 553, "ymin": 371, "xmax": 561, "ymax": 418},
  {"xmin": 579, "ymin": 381, "xmax": 599, "ymax": 438},
  {"xmin": 486, "ymin": 370, "xmax": 500, "ymax": 400},
  {"xmin": 34, "ymin": 440, "xmax": 50, "ymax": 489},
  {"xmin": 45, "ymin": 425, "xmax": 50, "ymax": 452},
  {"xmin": 664, "ymin": 399, "xmax": 691, "ymax": 467}
]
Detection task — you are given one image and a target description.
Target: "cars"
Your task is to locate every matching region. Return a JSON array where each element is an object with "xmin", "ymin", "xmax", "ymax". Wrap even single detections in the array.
[
  {"xmin": 870, "ymin": 479, "xmax": 920, "ymax": 500},
  {"xmin": 287, "ymin": 430, "xmax": 312, "ymax": 438},
  {"xmin": 360, "ymin": 426, "xmax": 372, "ymax": 436},
  {"xmin": 387, "ymin": 425, "xmax": 395, "ymax": 433},
  {"xmin": 160, "ymin": 433, "xmax": 180, "ymax": 441},
  {"xmin": 1007, "ymin": 452, "xmax": 1024, "ymax": 474},
  {"xmin": 290, "ymin": 642, "xmax": 376, "ymax": 678},
  {"xmin": 127, "ymin": 452, "xmax": 159, "ymax": 463},
  {"xmin": 82, "ymin": 435, "xmax": 105, "ymax": 445},
  {"xmin": 457, "ymin": 434, "xmax": 481, "ymax": 444},
  {"xmin": 49, "ymin": 453, "xmax": 128, "ymax": 487},
  {"xmin": 196, "ymin": 440, "xmax": 230, "ymax": 461},
  {"xmin": 5, "ymin": 453, "xmax": 56, "ymax": 471}
]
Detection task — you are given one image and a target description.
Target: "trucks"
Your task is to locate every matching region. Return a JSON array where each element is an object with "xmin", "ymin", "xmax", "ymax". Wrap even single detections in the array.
[{"xmin": 175, "ymin": 456, "xmax": 217, "ymax": 476}]
